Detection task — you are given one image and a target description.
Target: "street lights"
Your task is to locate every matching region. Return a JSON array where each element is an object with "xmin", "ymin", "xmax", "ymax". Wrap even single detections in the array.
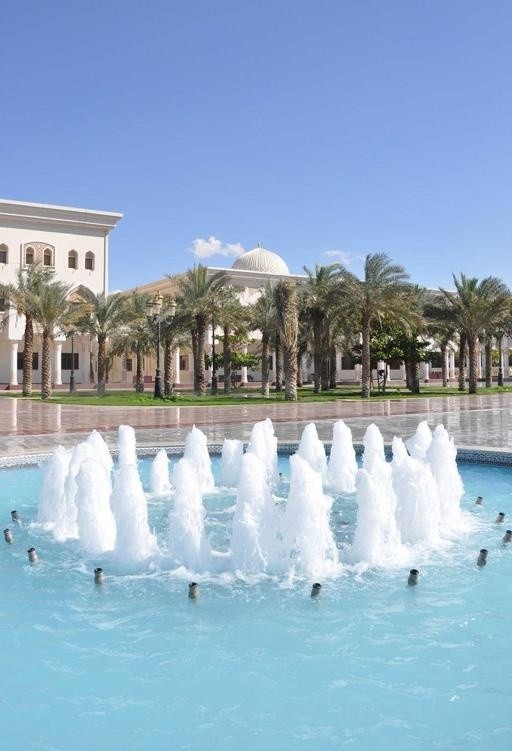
[
  {"xmin": 209, "ymin": 298, "xmax": 218, "ymax": 395},
  {"xmin": 494, "ymin": 328, "xmax": 505, "ymax": 387},
  {"xmin": 144, "ymin": 290, "xmax": 177, "ymax": 400},
  {"xmin": 69, "ymin": 331, "xmax": 79, "ymax": 393}
]
